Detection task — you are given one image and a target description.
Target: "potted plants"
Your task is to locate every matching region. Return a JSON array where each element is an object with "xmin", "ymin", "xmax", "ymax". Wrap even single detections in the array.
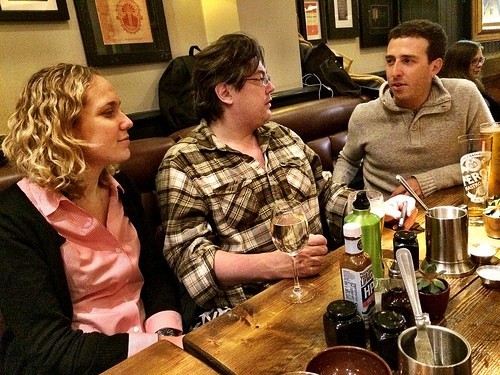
[{"xmin": 414, "ymin": 260, "xmax": 450, "ymax": 319}]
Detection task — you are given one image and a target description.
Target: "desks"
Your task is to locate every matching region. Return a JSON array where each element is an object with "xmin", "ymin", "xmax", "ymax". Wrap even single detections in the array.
[{"xmin": 184, "ymin": 187, "xmax": 500, "ymax": 375}]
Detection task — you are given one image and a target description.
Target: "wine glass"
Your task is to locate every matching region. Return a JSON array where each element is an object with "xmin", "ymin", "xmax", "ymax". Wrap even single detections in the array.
[{"xmin": 270, "ymin": 201, "xmax": 316, "ymax": 304}]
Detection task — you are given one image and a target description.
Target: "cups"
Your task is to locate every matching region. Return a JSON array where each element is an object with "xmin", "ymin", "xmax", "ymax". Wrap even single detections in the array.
[
  {"xmin": 419, "ymin": 206, "xmax": 474, "ymax": 277},
  {"xmin": 458, "ymin": 122, "xmax": 499, "ymax": 240},
  {"xmin": 398, "ymin": 325, "xmax": 471, "ymax": 375},
  {"xmin": 347, "ymin": 189, "xmax": 385, "ymax": 235}
]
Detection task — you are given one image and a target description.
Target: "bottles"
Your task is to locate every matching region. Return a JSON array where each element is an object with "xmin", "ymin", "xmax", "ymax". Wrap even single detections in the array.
[{"xmin": 323, "ymin": 191, "xmax": 419, "ymax": 369}]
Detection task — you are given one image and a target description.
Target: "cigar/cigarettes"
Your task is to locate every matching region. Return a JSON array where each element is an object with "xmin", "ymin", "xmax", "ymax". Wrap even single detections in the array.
[{"xmin": 398, "ymin": 201, "xmax": 408, "ymax": 227}]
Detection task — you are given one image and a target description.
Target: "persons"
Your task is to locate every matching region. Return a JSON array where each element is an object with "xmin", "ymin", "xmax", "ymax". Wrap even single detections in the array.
[
  {"xmin": 155, "ymin": 34, "xmax": 419, "ymax": 315},
  {"xmin": 440, "ymin": 40, "xmax": 500, "ymax": 123},
  {"xmin": 333, "ymin": 20, "xmax": 496, "ymax": 199},
  {"xmin": 0, "ymin": 64, "xmax": 186, "ymax": 375}
]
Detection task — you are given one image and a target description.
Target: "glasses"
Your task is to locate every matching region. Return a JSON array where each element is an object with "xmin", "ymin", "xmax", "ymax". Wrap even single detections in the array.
[
  {"xmin": 470, "ymin": 57, "xmax": 486, "ymax": 67},
  {"xmin": 244, "ymin": 75, "xmax": 271, "ymax": 86}
]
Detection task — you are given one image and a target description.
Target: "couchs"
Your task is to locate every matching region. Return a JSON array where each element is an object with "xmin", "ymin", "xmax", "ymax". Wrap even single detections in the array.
[{"xmin": 0, "ymin": 95, "xmax": 371, "ymax": 230}]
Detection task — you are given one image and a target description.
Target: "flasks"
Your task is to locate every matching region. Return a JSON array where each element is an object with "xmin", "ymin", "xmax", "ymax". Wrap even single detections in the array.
[{"xmin": 345, "ymin": 191, "xmax": 383, "ymax": 285}]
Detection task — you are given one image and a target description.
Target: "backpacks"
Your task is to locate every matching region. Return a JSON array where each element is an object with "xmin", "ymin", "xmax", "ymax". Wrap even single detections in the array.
[{"xmin": 158, "ymin": 45, "xmax": 202, "ymax": 136}]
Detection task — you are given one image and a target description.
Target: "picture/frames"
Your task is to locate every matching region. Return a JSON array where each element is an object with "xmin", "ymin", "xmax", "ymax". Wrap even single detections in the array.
[
  {"xmin": 297, "ymin": 0, "xmax": 327, "ymax": 46},
  {"xmin": 471, "ymin": 0, "xmax": 500, "ymax": 44},
  {"xmin": 327, "ymin": 0, "xmax": 359, "ymax": 39},
  {"xmin": 0, "ymin": 0, "xmax": 70, "ymax": 21},
  {"xmin": 358, "ymin": 0, "xmax": 398, "ymax": 49},
  {"xmin": 73, "ymin": 0, "xmax": 172, "ymax": 68}
]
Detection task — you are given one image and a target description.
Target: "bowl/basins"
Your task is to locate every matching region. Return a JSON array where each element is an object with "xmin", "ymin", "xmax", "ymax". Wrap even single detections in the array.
[
  {"xmin": 304, "ymin": 346, "xmax": 392, "ymax": 375},
  {"xmin": 469, "ymin": 246, "xmax": 500, "ymax": 288}
]
